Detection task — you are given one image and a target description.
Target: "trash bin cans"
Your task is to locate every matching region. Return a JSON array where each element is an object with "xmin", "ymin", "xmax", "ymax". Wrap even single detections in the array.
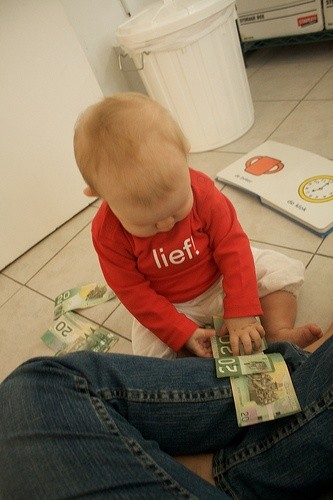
[{"xmin": 113, "ymin": 0, "xmax": 255, "ymax": 154}]
